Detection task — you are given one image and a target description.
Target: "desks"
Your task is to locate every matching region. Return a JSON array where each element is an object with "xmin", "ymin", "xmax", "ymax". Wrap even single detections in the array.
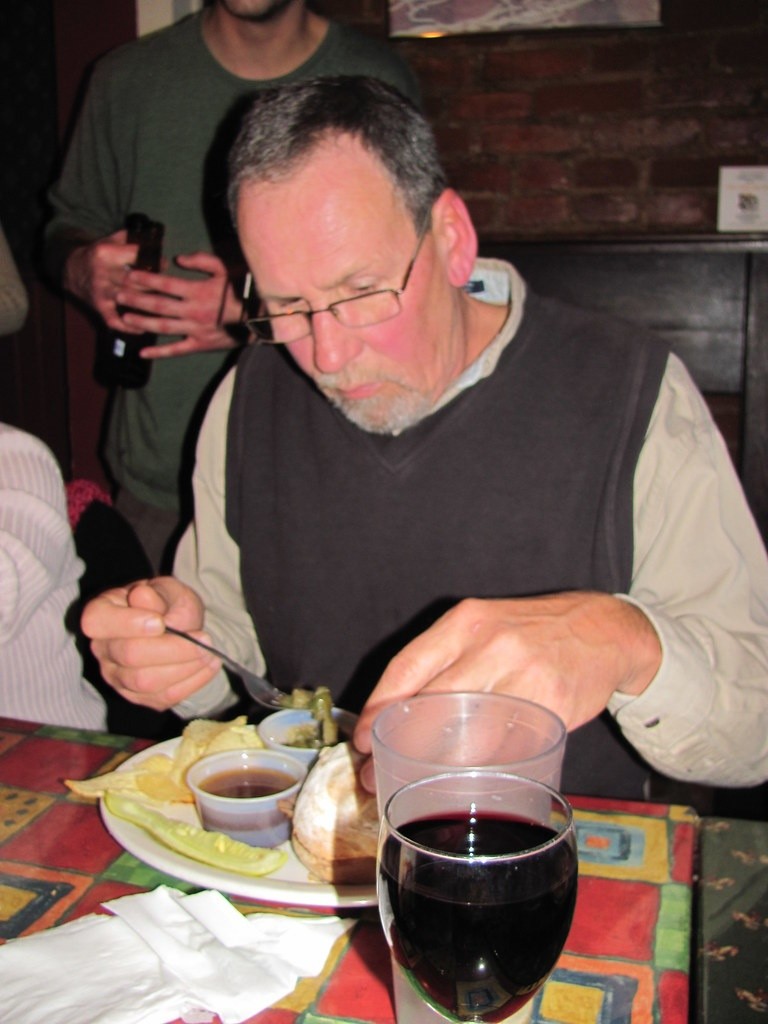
[{"xmin": 480, "ymin": 232, "xmax": 768, "ymax": 546}]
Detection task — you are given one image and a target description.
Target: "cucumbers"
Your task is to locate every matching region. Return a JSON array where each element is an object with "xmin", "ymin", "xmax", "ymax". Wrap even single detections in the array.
[{"xmin": 104, "ymin": 792, "xmax": 290, "ymax": 877}]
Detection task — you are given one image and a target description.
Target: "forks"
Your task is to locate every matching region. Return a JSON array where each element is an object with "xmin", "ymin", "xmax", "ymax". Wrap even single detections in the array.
[{"xmin": 164, "ymin": 623, "xmax": 291, "ymax": 711}]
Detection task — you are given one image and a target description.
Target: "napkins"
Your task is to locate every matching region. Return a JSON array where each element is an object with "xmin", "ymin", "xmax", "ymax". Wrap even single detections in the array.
[{"xmin": 0, "ymin": 882, "xmax": 359, "ymax": 1024}]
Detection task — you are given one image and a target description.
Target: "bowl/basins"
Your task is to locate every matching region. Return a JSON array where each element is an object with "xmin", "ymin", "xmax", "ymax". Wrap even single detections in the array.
[
  {"xmin": 257, "ymin": 706, "xmax": 360, "ymax": 769},
  {"xmin": 186, "ymin": 748, "xmax": 310, "ymax": 847}
]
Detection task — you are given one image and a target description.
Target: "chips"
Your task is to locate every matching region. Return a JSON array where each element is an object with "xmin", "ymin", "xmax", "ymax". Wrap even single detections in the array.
[{"xmin": 62, "ymin": 714, "xmax": 265, "ymax": 803}]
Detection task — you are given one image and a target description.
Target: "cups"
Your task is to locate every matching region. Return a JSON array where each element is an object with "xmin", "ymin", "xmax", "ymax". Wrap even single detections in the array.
[{"xmin": 372, "ymin": 691, "xmax": 569, "ymax": 824}]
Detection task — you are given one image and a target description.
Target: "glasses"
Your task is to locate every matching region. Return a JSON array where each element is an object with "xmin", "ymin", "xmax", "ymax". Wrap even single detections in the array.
[{"xmin": 241, "ymin": 208, "xmax": 431, "ymax": 344}]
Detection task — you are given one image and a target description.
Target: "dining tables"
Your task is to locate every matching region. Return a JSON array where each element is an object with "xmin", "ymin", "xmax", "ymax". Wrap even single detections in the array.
[{"xmin": 0, "ymin": 717, "xmax": 700, "ymax": 1024}]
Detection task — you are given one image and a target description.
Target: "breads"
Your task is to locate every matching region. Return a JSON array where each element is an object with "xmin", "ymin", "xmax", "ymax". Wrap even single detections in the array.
[{"xmin": 289, "ymin": 741, "xmax": 386, "ymax": 884}]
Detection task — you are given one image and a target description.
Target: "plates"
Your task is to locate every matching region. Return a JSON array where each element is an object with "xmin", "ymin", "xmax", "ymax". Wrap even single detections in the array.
[{"xmin": 99, "ymin": 722, "xmax": 380, "ymax": 908}]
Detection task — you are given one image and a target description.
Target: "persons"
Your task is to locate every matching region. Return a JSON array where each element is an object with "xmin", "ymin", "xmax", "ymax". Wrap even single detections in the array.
[
  {"xmin": 80, "ymin": 73, "xmax": 768, "ymax": 800},
  {"xmin": 42, "ymin": 1, "xmax": 423, "ymax": 572},
  {"xmin": 0, "ymin": 228, "xmax": 106, "ymax": 733}
]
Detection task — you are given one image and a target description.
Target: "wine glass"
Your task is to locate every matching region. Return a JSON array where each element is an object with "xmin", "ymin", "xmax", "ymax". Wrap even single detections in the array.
[{"xmin": 376, "ymin": 771, "xmax": 578, "ymax": 1024}]
